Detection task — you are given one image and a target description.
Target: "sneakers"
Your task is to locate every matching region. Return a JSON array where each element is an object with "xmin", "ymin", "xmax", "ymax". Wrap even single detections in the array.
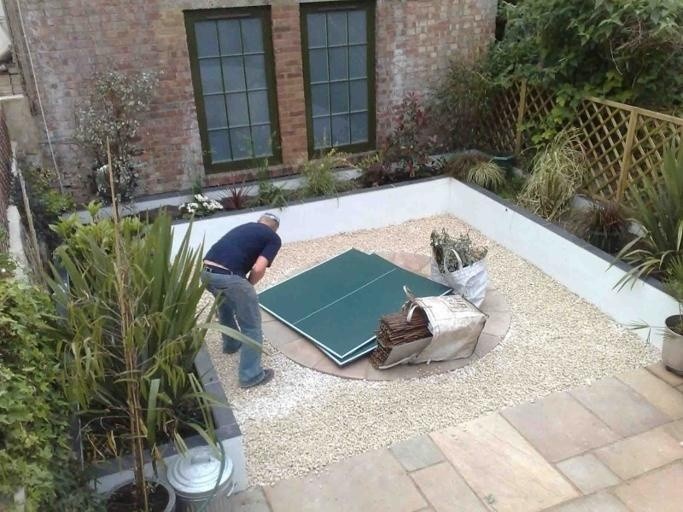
[{"xmin": 242, "ymin": 369, "xmax": 274, "ymax": 388}]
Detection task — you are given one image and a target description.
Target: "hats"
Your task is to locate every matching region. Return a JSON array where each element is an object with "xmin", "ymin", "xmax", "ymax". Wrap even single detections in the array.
[{"xmin": 264, "ymin": 211, "xmax": 280, "ymax": 223}]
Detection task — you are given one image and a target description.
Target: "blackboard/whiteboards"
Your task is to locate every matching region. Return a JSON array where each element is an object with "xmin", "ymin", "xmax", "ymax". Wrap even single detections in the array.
[{"xmin": 256, "ymin": 246, "xmax": 455, "ymax": 367}]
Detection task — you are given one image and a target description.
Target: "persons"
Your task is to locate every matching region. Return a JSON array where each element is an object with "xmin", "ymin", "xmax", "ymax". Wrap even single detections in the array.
[{"xmin": 194, "ymin": 210, "xmax": 282, "ymax": 390}]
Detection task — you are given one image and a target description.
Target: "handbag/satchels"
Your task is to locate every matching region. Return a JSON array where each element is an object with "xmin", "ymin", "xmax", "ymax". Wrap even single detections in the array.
[
  {"xmin": 403, "ymin": 295, "xmax": 488, "ymax": 364},
  {"xmin": 431, "ymin": 248, "xmax": 487, "ymax": 308}
]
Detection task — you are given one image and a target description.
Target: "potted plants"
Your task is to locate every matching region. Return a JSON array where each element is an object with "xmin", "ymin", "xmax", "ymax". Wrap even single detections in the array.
[
  {"xmin": 100, "ymin": 137, "xmax": 176, "ymax": 512},
  {"xmin": 606, "ymin": 135, "xmax": 683, "ymax": 377}
]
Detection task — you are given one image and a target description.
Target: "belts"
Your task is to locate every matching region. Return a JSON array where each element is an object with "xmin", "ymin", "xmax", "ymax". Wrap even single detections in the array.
[{"xmin": 201, "ymin": 267, "xmax": 241, "ymax": 274}]
